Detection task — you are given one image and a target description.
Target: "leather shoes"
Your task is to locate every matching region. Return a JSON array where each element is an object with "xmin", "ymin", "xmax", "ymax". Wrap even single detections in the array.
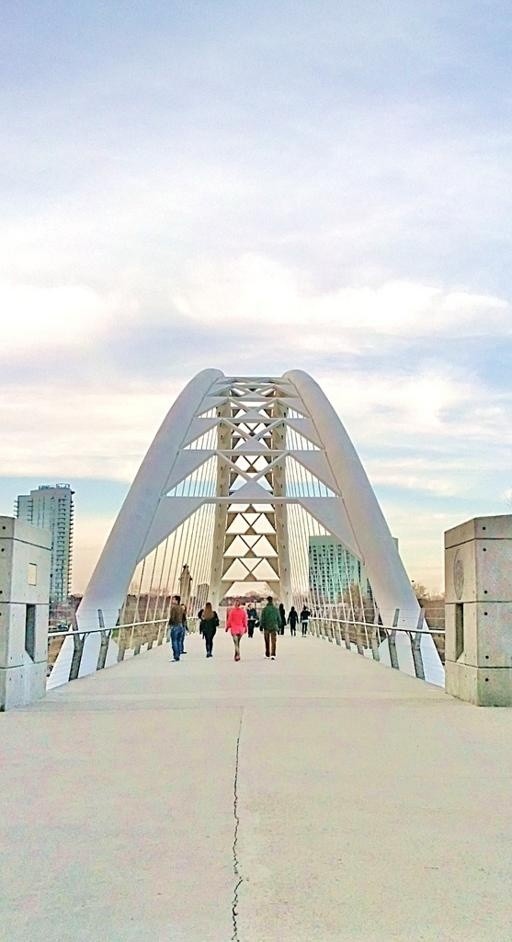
[{"xmin": 206, "ymin": 652, "xmax": 212, "ymax": 658}]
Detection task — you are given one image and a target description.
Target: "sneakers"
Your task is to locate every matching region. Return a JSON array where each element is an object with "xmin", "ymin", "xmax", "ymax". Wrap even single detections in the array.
[
  {"xmin": 271, "ymin": 656, "xmax": 275, "ymax": 660},
  {"xmin": 302, "ymin": 634, "xmax": 306, "ymax": 637},
  {"xmin": 264, "ymin": 656, "xmax": 270, "ymax": 660},
  {"xmin": 170, "ymin": 651, "xmax": 186, "ymax": 662},
  {"xmin": 234, "ymin": 655, "xmax": 241, "ymax": 662}
]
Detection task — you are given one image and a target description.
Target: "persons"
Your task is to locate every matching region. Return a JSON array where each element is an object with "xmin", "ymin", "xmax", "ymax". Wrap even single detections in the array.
[
  {"xmin": 287, "ymin": 606, "xmax": 299, "ymax": 637},
  {"xmin": 259, "ymin": 595, "xmax": 282, "ymax": 660},
  {"xmin": 277, "ymin": 604, "xmax": 287, "ymax": 635},
  {"xmin": 198, "ymin": 601, "xmax": 219, "ymax": 659},
  {"xmin": 168, "ymin": 595, "xmax": 184, "ymax": 663},
  {"xmin": 299, "ymin": 604, "xmax": 311, "ymax": 637},
  {"xmin": 246, "ymin": 603, "xmax": 259, "ymax": 639},
  {"xmin": 225, "ymin": 600, "xmax": 248, "ymax": 661},
  {"xmin": 180, "ymin": 604, "xmax": 190, "ymax": 654}
]
